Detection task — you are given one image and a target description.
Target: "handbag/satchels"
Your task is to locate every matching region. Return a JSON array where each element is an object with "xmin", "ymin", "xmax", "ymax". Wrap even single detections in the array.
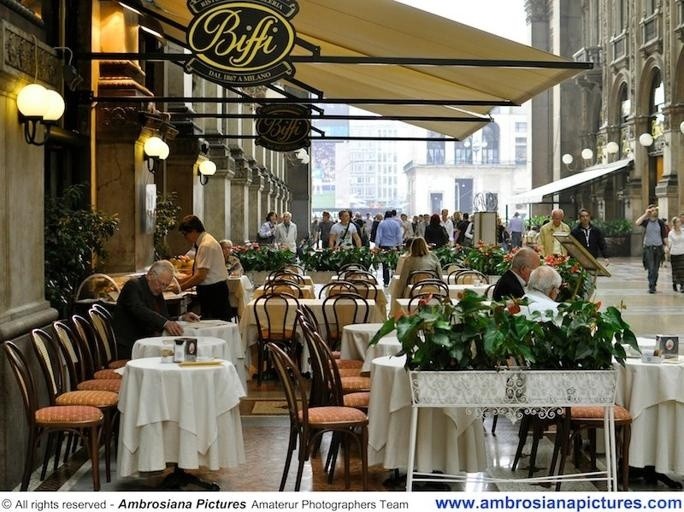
[{"xmin": 255, "ymin": 233, "xmax": 275, "ymax": 245}]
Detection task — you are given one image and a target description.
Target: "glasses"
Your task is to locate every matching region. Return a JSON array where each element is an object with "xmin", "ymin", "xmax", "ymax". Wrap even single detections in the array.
[{"xmin": 157, "ymin": 279, "xmax": 166, "ymax": 289}]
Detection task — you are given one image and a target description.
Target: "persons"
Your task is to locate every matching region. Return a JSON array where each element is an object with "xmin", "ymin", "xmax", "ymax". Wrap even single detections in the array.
[
  {"xmin": 662, "ymin": 218, "xmax": 670, "ymax": 268},
  {"xmin": 400, "ymin": 238, "xmax": 443, "ymax": 299},
  {"xmin": 504, "ymin": 265, "xmax": 569, "ymax": 327},
  {"xmin": 636, "ymin": 204, "xmax": 666, "ymax": 293},
  {"xmin": 490, "ymin": 247, "xmax": 540, "ymax": 315},
  {"xmin": 312, "ymin": 208, "xmax": 611, "ymax": 269},
  {"xmin": 114, "ymin": 260, "xmax": 201, "ymax": 356},
  {"xmin": 275, "ymin": 211, "xmax": 297, "ymax": 253},
  {"xmin": 171, "ymin": 216, "xmax": 233, "ymax": 322},
  {"xmin": 258, "ymin": 211, "xmax": 278, "ymax": 248},
  {"xmin": 668, "ymin": 217, "xmax": 684, "ymax": 293},
  {"xmin": 679, "ymin": 213, "xmax": 684, "ymax": 228},
  {"xmin": 218, "ymin": 239, "xmax": 245, "ymax": 276}
]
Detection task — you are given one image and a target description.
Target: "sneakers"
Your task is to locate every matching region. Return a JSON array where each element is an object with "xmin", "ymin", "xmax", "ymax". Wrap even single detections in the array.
[{"xmin": 648, "ymin": 286, "xmax": 656, "ymax": 293}]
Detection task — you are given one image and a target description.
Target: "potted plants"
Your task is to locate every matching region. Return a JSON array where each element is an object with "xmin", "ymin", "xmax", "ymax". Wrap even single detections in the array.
[{"xmin": 595, "ymin": 217, "xmax": 633, "ymax": 258}]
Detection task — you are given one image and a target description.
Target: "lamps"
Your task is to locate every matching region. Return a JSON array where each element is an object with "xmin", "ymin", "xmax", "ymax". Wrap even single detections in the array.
[
  {"xmin": 606, "ymin": 142, "xmax": 619, "ymax": 162},
  {"xmin": 562, "ymin": 153, "xmax": 577, "ymax": 171},
  {"xmin": 581, "ymin": 148, "xmax": 595, "ymax": 167},
  {"xmin": 284, "ymin": 147, "xmax": 310, "ymax": 169},
  {"xmin": 639, "ymin": 133, "xmax": 656, "ymax": 153},
  {"xmin": 143, "ymin": 137, "xmax": 170, "ymax": 175},
  {"xmin": 17, "ymin": 84, "xmax": 66, "ymax": 145},
  {"xmin": 192, "ymin": 138, "xmax": 216, "ymax": 186}
]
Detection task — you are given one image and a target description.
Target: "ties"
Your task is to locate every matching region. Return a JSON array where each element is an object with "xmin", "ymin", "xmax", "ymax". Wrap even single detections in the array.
[{"xmin": 193, "ymin": 242, "xmax": 198, "ymax": 275}]
